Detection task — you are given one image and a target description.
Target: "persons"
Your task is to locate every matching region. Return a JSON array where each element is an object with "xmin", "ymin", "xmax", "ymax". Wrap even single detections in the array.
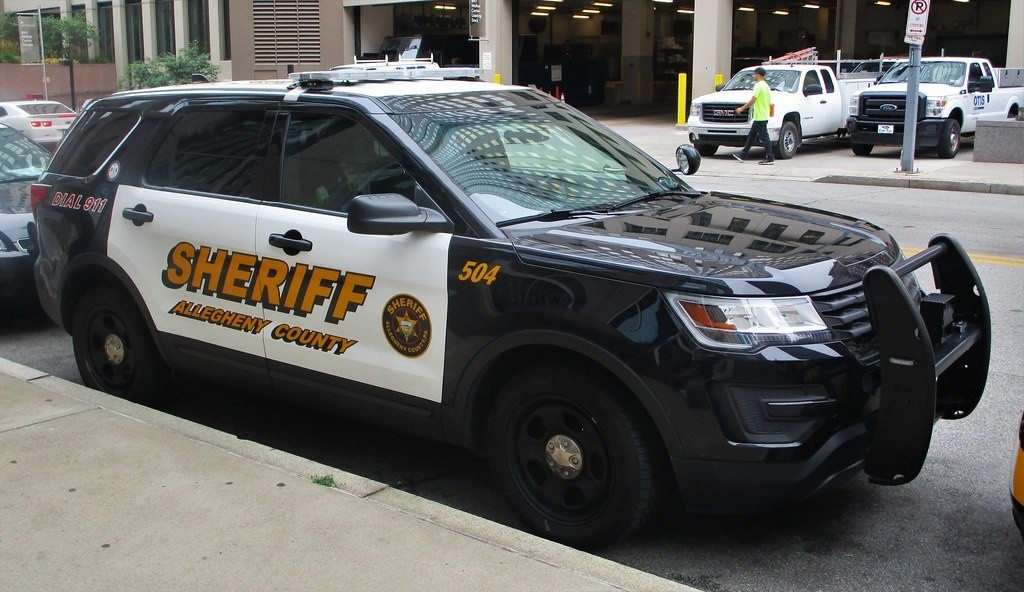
[{"xmin": 732, "ymin": 68, "xmax": 775, "ymax": 164}]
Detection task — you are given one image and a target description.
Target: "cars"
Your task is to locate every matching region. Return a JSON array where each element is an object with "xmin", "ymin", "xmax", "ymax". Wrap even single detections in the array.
[
  {"xmin": 0, "ymin": 101, "xmax": 77, "ymax": 143},
  {"xmin": 0, "ymin": 121, "xmax": 54, "ymax": 334}
]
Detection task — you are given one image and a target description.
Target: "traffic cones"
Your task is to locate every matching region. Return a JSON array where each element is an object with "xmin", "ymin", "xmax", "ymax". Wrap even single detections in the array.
[
  {"xmin": 548, "ymin": 91, "xmax": 552, "ymax": 96},
  {"xmin": 560, "ymin": 92, "xmax": 566, "ymax": 104}
]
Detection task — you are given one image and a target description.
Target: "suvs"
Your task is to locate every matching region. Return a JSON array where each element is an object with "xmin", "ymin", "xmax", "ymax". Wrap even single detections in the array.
[{"xmin": 26, "ymin": 52, "xmax": 993, "ymax": 558}]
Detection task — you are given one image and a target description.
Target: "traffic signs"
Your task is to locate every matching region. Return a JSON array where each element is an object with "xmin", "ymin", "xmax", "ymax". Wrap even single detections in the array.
[{"xmin": 906, "ymin": 0, "xmax": 930, "ymax": 36}]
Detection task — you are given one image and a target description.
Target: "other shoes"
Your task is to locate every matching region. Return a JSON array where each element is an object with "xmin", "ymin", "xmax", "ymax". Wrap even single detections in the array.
[
  {"xmin": 732, "ymin": 152, "xmax": 744, "ymax": 163},
  {"xmin": 758, "ymin": 159, "xmax": 775, "ymax": 165}
]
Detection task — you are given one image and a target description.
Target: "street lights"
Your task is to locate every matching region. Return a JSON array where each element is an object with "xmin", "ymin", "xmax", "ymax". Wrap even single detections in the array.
[{"xmin": 58, "ymin": 58, "xmax": 75, "ymax": 111}]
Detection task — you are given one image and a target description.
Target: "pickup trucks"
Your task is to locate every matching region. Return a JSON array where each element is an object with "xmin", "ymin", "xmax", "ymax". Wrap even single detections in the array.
[
  {"xmin": 851, "ymin": 56, "xmax": 899, "ymax": 74},
  {"xmin": 687, "ymin": 65, "xmax": 877, "ymax": 160},
  {"xmin": 845, "ymin": 58, "xmax": 1024, "ymax": 158}
]
